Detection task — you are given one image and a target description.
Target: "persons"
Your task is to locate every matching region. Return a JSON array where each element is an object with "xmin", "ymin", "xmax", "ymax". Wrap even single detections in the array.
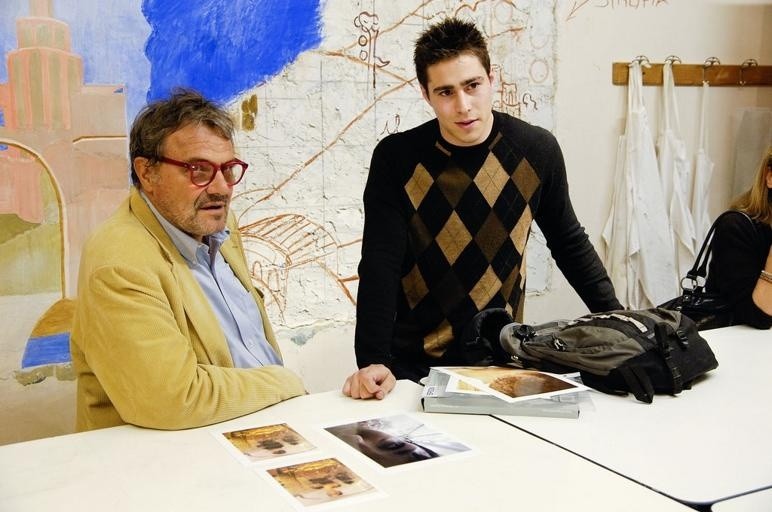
[
  {"xmin": 70, "ymin": 86, "xmax": 308, "ymax": 432},
  {"xmin": 702, "ymin": 152, "xmax": 772, "ymax": 330},
  {"xmin": 340, "ymin": 13, "xmax": 625, "ymax": 400},
  {"xmin": 357, "ymin": 429, "xmax": 439, "ymax": 467}
]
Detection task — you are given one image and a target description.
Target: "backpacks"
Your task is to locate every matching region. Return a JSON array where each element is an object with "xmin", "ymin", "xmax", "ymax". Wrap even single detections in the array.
[{"xmin": 461, "ymin": 306, "xmax": 719, "ymax": 403}]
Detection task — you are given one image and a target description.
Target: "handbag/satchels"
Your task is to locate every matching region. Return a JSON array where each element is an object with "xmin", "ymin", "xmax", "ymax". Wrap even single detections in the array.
[{"xmin": 655, "ymin": 295, "xmax": 727, "ymax": 329}]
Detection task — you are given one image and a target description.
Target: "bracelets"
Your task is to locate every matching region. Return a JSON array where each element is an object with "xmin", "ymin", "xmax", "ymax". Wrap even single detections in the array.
[{"xmin": 759, "ymin": 270, "xmax": 772, "ymax": 285}]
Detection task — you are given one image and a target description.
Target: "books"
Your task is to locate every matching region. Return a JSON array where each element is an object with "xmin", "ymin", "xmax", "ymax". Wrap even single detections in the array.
[{"xmin": 421, "ymin": 366, "xmax": 579, "ymax": 417}]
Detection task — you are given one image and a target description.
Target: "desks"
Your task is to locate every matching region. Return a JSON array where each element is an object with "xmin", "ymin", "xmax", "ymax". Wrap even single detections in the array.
[
  {"xmin": 711, "ymin": 487, "xmax": 771, "ymax": 511},
  {"xmin": 419, "ymin": 323, "xmax": 771, "ymax": 504},
  {"xmin": 3, "ymin": 377, "xmax": 696, "ymax": 511}
]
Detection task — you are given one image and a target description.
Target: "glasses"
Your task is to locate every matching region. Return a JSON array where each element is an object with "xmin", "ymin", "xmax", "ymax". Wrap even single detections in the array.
[{"xmin": 155, "ymin": 155, "xmax": 248, "ymax": 188}]
